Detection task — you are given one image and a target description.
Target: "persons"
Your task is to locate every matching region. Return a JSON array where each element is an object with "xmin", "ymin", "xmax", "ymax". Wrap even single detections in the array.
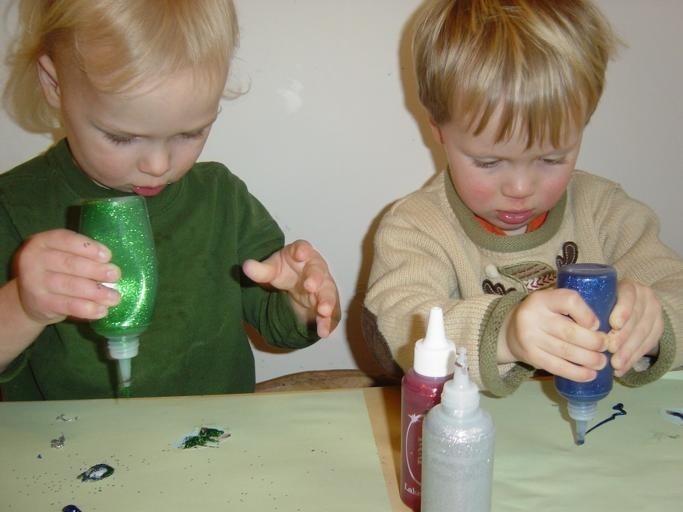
[
  {"xmin": 362, "ymin": 0, "xmax": 682, "ymax": 396},
  {"xmin": 0, "ymin": 0, "xmax": 338, "ymax": 399}
]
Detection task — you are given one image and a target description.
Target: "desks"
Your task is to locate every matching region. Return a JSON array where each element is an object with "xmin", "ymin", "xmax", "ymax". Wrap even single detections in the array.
[{"xmin": 0, "ymin": 370, "xmax": 683, "ymax": 512}]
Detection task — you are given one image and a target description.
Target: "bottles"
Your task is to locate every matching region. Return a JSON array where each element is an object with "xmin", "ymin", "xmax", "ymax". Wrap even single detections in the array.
[
  {"xmin": 420, "ymin": 347, "xmax": 494, "ymax": 512},
  {"xmin": 78, "ymin": 196, "xmax": 157, "ymax": 388},
  {"xmin": 556, "ymin": 264, "xmax": 616, "ymax": 444},
  {"xmin": 399, "ymin": 306, "xmax": 457, "ymax": 512}
]
